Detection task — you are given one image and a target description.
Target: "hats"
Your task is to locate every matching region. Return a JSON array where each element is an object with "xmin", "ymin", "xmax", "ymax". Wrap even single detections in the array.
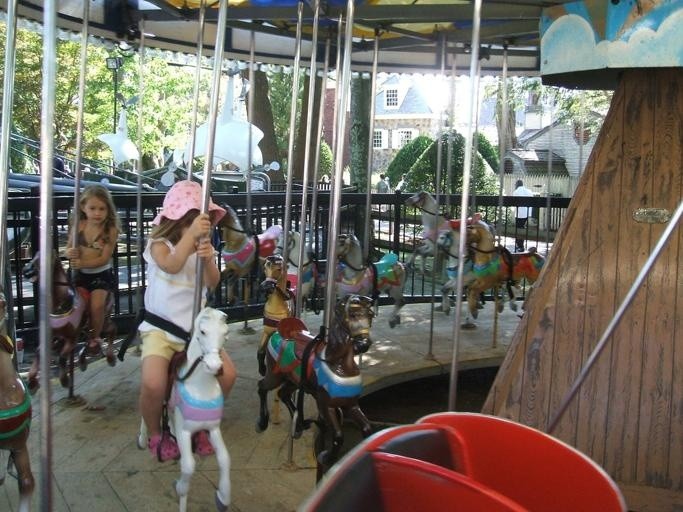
[{"xmin": 150, "ymin": 180, "xmax": 228, "ymax": 227}]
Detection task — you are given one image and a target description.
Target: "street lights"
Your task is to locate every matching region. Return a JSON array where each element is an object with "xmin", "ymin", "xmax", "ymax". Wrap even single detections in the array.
[{"xmin": 106, "ymin": 57, "xmax": 123, "ymax": 135}]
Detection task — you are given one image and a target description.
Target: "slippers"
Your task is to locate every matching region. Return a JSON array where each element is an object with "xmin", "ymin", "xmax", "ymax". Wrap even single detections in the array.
[
  {"xmin": 148, "ymin": 433, "xmax": 180, "ymax": 460},
  {"xmin": 192, "ymin": 431, "xmax": 214, "ymax": 455}
]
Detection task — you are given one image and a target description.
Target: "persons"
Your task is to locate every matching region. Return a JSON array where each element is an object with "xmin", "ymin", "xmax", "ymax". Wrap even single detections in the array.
[
  {"xmin": 376, "ymin": 173, "xmax": 390, "ymax": 193},
  {"xmin": 395, "ymin": 173, "xmax": 408, "ymax": 192},
  {"xmin": 137, "ymin": 180, "xmax": 237, "ymax": 461},
  {"xmin": 62, "ymin": 184, "xmax": 119, "ymax": 358},
  {"xmin": 510, "ymin": 179, "xmax": 534, "ymax": 253}
]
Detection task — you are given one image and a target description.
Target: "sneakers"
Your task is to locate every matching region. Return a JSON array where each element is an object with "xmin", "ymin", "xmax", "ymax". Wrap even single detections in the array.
[{"xmin": 88, "ymin": 342, "xmax": 101, "ymax": 355}]
